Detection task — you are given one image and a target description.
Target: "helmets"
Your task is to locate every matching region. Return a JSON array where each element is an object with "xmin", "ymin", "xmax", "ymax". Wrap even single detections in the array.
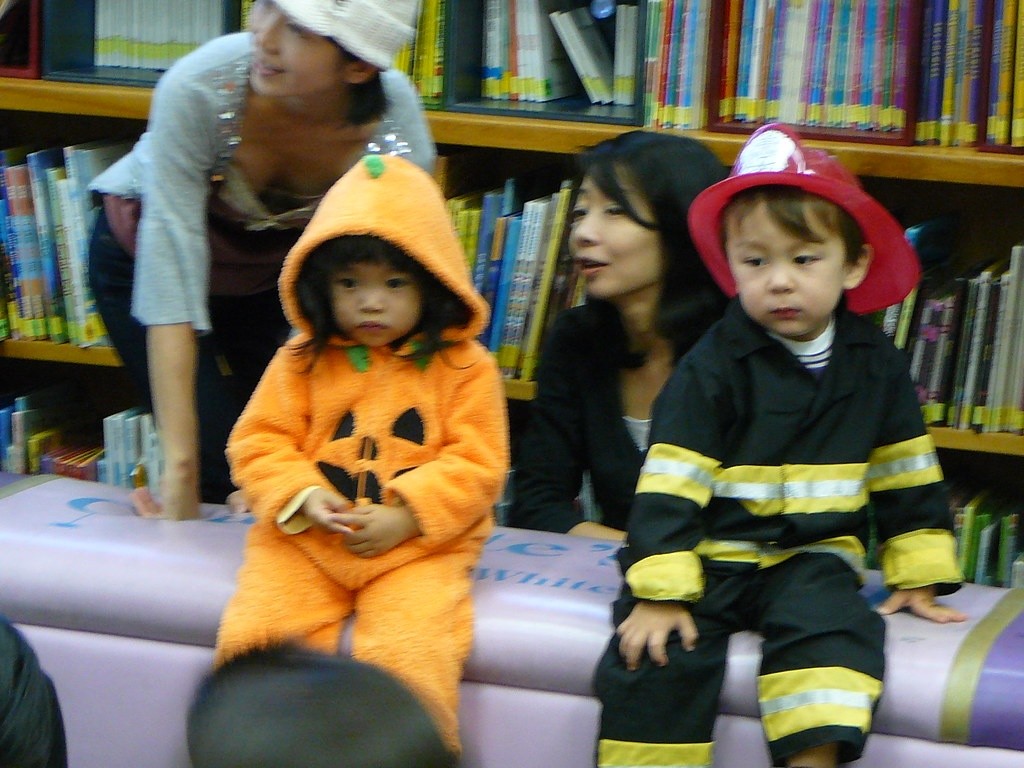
[{"xmin": 689, "ymin": 124, "xmax": 920, "ymax": 314}]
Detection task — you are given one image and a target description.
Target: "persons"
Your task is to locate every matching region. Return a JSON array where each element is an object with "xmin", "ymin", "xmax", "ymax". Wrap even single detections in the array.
[
  {"xmin": 210, "ymin": 154, "xmax": 509, "ymax": 756},
  {"xmin": 511, "ymin": 131, "xmax": 731, "ymax": 544},
  {"xmin": 592, "ymin": 123, "xmax": 971, "ymax": 768},
  {"xmin": 183, "ymin": 635, "xmax": 457, "ymax": 768},
  {"xmin": 84, "ymin": 0, "xmax": 440, "ymax": 522},
  {"xmin": 0, "ymin": 614, "xmax": 68, "ymax": 768}
]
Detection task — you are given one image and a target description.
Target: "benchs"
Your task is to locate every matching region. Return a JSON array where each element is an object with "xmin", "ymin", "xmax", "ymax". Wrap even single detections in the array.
[{"xmin": 0, "ymin": 467, "xmax": 1023, "ymax": 768}]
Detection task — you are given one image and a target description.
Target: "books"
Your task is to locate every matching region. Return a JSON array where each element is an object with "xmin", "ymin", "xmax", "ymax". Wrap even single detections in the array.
[
  {"xmin": 866, "ymin": 470, "xmax": 1024, "ymax": 589},
  {"xmin": 481, "ymin": 0, "xmax": 638, "ymax": 108},
  {"xmin": 239, "ymin": 0, "xmax": 443, "ymax": 110},
  {"xmin": 861, "ymin": 219, "xmax": 1024, "ymax": 437},
  {"xmin": 0, "ymin": 395, "xmax": 162, "ymax": 491},
  {"xmin": 644, "ymin": 0, "xmax": 1024, "ymax": 150},
  {"xmin": 91, "ymin": 0, "xmax": 224, "ymax": 71},
  {"xmin": 443, "ymin": 174, "xmax": 589, "ymax": 381},
  {"xmin": 0, "ymin": 140, "xmax": 112, "ymax": 346}
]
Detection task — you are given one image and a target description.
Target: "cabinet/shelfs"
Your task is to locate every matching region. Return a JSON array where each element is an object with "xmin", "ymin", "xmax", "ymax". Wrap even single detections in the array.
[{"xmin": 0, "ymin": 68, "xmax": 1024, "ymax": 467}]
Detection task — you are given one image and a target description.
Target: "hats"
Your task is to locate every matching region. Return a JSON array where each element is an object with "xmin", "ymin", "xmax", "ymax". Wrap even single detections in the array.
[{"xmin": 272, "ymin": 0, "xmax": 419, "ymax": 70}]
